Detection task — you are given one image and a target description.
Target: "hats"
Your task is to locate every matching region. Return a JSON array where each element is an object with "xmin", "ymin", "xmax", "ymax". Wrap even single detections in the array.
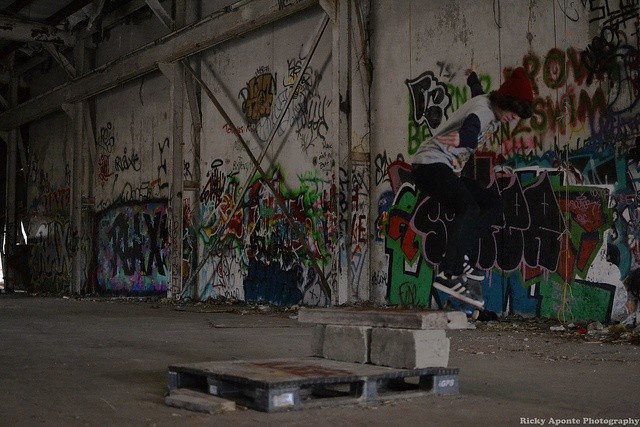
[{"xmin": 498, "ymin": 66, "xmax": 533, "ymax": 101}]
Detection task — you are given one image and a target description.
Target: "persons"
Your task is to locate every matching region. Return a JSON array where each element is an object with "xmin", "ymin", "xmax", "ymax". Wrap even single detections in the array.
[{"xmin": 409, "ymin": 66, "xmax": 535, "ymax": 307}]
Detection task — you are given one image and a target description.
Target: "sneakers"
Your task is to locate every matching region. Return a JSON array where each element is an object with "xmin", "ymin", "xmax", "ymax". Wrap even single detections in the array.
[
  {"xmin": 433, "ymin": 271, "xmax": 485, "ymax": 308},
  {"xmin": 461, "ymin": 255, "xmax": 486, "ymax": 281}
]
